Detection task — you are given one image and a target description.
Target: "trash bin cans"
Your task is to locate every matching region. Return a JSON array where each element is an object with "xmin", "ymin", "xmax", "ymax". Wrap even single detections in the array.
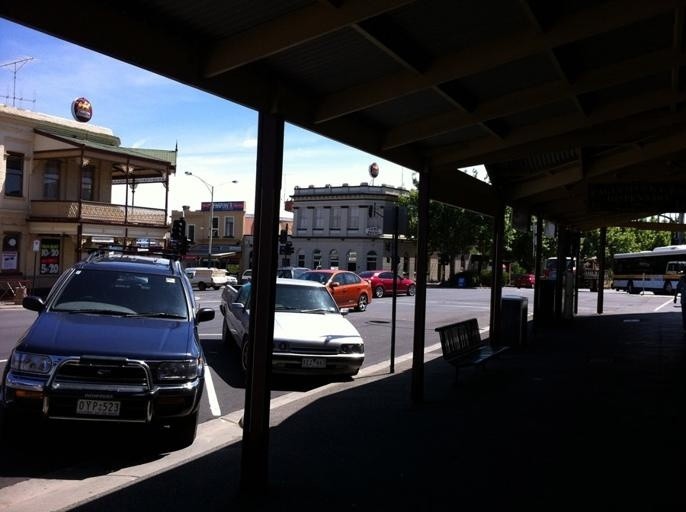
[
  {"xmin": 501, "ymin": 295, "xmax": 529, "ymax": 327},
  {"xmin": 15, "ymin": 288, "xmax": 27, "ymax": 305}
]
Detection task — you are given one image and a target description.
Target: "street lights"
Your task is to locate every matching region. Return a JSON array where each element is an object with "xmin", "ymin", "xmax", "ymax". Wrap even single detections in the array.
[{"xmin": 185, "ymin": 171, "xmax": 214, "ymax": 269}]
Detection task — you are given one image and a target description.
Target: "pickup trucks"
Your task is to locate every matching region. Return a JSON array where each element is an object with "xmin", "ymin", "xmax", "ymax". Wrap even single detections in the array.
[{"xmin": 220, "ymin": 278, "xmax": 364, "ymax": 381}]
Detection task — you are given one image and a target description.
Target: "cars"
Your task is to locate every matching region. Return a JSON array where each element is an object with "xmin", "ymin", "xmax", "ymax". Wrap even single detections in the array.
[
  {"xmin": 516, "ymin": 274, "xmax": 535, "ymax": 288},
  {"xmin": 276, "ymin": 267, "xmax": 310, "ymax": 279},
  {"xmin": 240, "ymin": 269, "xmax": 252, "ymax": 283},
  {"xmin": 296, "ymin": 269, "xmax": 372, "ymax": 312},
  {"xmin": 355, "ymin": 270, "xmax": 417, "ymax": 298}
]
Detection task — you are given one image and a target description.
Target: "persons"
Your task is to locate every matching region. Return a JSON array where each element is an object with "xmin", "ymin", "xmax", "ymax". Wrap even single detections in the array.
[{"xmin": 673, "ymin": 272, "xmax": 685, "ymax": 328}]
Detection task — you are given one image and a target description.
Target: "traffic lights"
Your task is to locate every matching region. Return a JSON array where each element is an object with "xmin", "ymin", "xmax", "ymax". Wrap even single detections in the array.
[
  {"xmin": 170, "ymin": 219, "xmax": 177, "ymax": 240},
  {"xmin": 285, "ymin": 241, "xmax": 294, "ymax": 255},
  {"xmin": 176, "ymin": 219, "xmax": 185, "ymax": 240}
]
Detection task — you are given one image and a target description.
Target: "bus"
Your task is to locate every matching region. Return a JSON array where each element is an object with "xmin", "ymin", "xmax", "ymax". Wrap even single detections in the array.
[{"xmin": 610, "ymin": 244, "xmax": 686, "ymax": 295}]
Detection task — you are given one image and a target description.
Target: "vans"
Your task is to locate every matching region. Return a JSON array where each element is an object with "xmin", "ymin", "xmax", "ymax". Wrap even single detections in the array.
[{"xmin": 666, "ymin": 262, "xmax": 685, "ymax": 274}]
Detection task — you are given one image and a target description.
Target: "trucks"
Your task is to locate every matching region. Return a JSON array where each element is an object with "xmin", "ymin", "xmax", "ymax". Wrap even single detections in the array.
[
  {"xmin": 545, "ymin": 255, "xmax": 609, "ymax": 292},
  {"xmin": 194, "ymin": 268, "xmax": 237, "ymax": 291},
  {"xmin": 183, "ymin": 267, "xmax": 218, "ymax": 287}
]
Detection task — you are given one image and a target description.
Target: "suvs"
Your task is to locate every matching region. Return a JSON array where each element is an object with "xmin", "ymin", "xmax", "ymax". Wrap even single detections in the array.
[{"xmin": 0, "ymin": 248, "xmax": 216, "ymax": 450}]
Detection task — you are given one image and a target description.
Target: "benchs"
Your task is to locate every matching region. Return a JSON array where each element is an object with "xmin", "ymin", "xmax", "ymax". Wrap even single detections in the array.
[{"xmin": 435, "ymin": 317, "xmax": 512, "ymax": 387}]
